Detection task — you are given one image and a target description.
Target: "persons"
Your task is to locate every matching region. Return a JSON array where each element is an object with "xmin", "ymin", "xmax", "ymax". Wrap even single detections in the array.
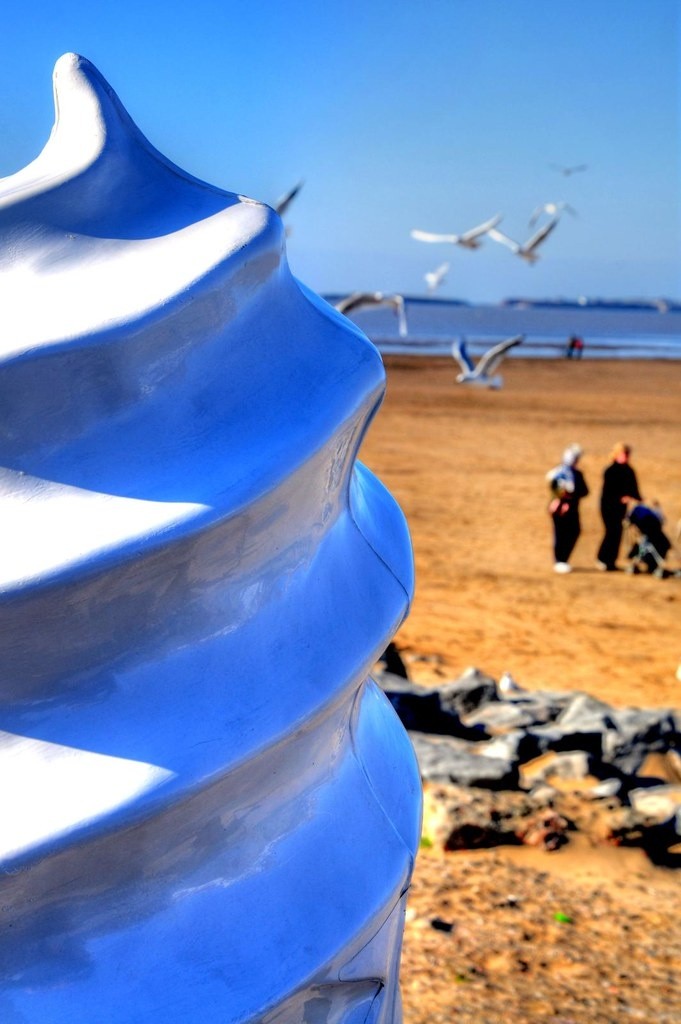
[
  {"xmin": 542, "ymin": 439, "xmax": 680, "ymax": 577},
  {"xmin": 566, "ymin": 333, "xmax": 584, "ymax": 357}
]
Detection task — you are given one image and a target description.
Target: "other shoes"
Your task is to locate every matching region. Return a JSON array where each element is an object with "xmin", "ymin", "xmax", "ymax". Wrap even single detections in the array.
[
  {"xmin": 559, "ymin": 503, "xmax": 569, "ymax": 516},
  {"xmin": 549, "ymin": 498, "xmax": 560, "ymax": 512},
  {"xmin": 554, "ymin": 562, "xmax": 571, "ymax": 573}
]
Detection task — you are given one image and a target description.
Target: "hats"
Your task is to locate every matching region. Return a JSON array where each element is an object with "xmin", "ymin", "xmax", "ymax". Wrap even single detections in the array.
[{"xmin": 563, "ymin": 450, "xmax": 575, "ymax": 465}]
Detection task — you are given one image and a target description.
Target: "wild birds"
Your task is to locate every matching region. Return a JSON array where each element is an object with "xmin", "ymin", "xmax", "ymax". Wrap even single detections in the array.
[{"xmin": 255, "ymin": 180, "xmax": 576, "ymax": 389}]
